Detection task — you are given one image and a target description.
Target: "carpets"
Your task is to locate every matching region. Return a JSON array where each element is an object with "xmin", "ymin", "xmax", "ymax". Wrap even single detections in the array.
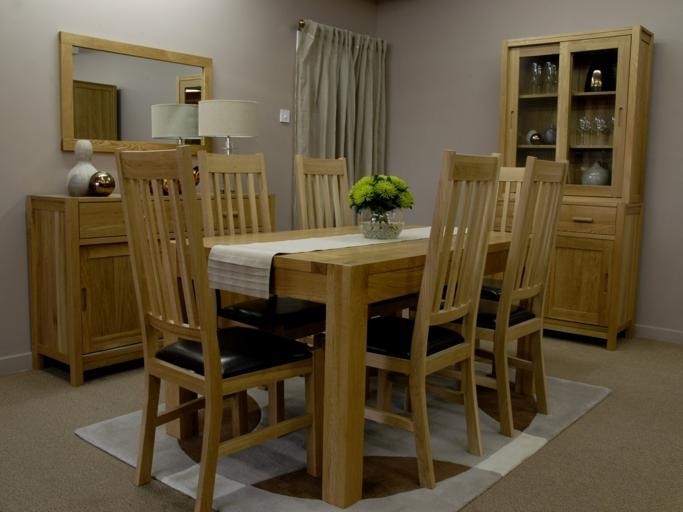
[{"xmin": 71, "ymin": 361, "xmax": 612, "ymax": 512}]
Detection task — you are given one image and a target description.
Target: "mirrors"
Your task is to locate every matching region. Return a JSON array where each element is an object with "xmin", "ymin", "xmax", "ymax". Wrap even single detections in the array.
[{"xmin": 59, "ymin": 31, "xmax": 214, "ymax": 158}]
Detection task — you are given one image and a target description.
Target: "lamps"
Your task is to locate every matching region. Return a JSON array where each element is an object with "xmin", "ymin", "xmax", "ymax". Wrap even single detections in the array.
[
  {"xmin": 151, "ymin": 103, "xmax": 204, "ymax": 144},
  {"xmin": 198, "ymin": 98, "xmax": 260, "ymax": 193}
]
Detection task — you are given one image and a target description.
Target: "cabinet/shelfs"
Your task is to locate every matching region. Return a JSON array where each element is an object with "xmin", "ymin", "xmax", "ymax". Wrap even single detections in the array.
[
  {"xmin": 24, "ymin": 192, "xmax": 276, "ymax": 387},
  {"xmin": 492, "ymin": 24, "xmax": 655, "ymax": 352}
]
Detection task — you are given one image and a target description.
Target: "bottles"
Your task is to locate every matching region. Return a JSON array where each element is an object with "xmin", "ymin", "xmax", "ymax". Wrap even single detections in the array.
[{"xmin": 524, "ymin": 61, "xmax": 613, "ymax": 146}]
